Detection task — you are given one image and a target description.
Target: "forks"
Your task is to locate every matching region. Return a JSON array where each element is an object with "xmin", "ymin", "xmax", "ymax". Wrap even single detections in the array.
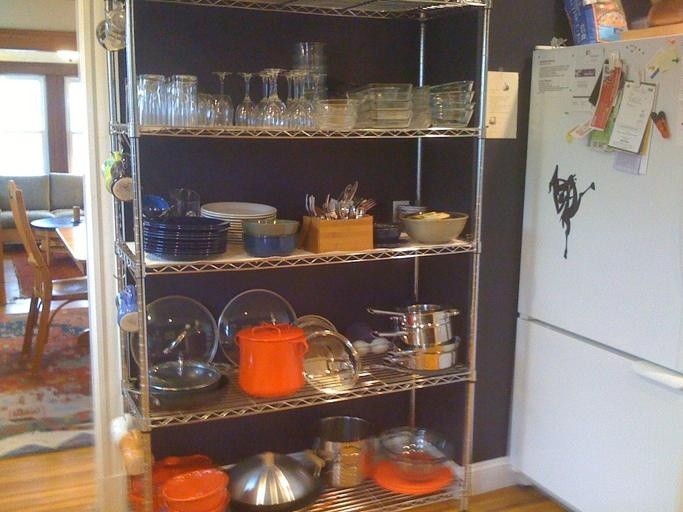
[{"xmin": 305, "ymin": 181, "xmax": 377, "ymax": 221}]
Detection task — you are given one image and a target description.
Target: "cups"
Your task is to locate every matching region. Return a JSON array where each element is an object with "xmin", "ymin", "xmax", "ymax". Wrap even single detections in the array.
[
  {"xmin": 375, "ymin": 206, "xmax": 427, "ymax": 244},
  {"xmin": 123, "ymin": 73, "xmax": 213, "ymax": 128}
]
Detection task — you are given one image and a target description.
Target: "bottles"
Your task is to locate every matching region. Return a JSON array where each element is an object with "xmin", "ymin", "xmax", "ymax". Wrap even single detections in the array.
[{"xmin": 73, "ymin": 206, "xmax": 81, "ymax": 222}]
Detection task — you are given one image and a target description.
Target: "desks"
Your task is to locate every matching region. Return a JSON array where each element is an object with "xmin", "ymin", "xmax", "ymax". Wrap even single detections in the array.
[{"xmin": 55, "ymin": 226, "xmax": 87, "ymax": 274}]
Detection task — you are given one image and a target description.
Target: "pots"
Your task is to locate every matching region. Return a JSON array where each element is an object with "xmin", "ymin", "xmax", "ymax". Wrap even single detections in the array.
[{"xmin": 125, "ymin": 286, "xmax": 460, "ymax": 411}]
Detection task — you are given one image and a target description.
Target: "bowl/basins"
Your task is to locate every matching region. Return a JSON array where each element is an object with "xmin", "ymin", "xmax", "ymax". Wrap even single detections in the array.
[
  {"xmin": 151, "ymin": 418, "xmax": 456, "ymax": 512},
  {"xmin": 400, "ymin": 211, "xmax": 469, "ymax": 244},
  {"xmin": 291, "ymin": 39, "xmax": 476, "ymax": 130}
]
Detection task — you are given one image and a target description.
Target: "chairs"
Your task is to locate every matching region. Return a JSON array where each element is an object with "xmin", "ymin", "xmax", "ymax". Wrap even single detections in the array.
[{"xmin": 8, "ymin": 179, "xmax": 87, "ymax": 374}]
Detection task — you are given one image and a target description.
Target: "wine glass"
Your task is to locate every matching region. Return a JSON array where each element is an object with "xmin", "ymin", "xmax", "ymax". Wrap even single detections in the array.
[{"xmin": 213, "ymin": 69, "xmax": 328, "ymax": 129}]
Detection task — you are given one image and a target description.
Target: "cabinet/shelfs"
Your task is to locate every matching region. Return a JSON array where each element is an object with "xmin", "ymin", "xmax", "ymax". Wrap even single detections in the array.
[{"xmin": 105, "ymin": 0, "xmax": 492, "ymax": 512}]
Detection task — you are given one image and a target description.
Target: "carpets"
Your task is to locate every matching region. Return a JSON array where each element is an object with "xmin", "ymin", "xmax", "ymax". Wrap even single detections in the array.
[{"xmin": 0, "ymin": 307, "xmax": 94, "ymax": 462}]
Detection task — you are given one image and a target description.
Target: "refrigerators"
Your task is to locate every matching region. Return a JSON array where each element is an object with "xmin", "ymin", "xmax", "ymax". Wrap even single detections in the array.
[{"xmin": 508, "ymin": 35, "xmax": 682, "ymax": 512}]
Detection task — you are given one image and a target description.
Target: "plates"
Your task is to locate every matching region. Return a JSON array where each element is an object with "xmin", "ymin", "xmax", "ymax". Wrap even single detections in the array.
[{"xmin": 142, "ymin": 186, "xmax": 299, "ymax": 262}]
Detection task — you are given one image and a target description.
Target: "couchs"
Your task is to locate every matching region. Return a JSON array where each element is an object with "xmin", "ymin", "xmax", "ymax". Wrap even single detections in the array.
[{"xmin": 0, "ymin": 173, "xmax": 85, "ymax": 246}]
